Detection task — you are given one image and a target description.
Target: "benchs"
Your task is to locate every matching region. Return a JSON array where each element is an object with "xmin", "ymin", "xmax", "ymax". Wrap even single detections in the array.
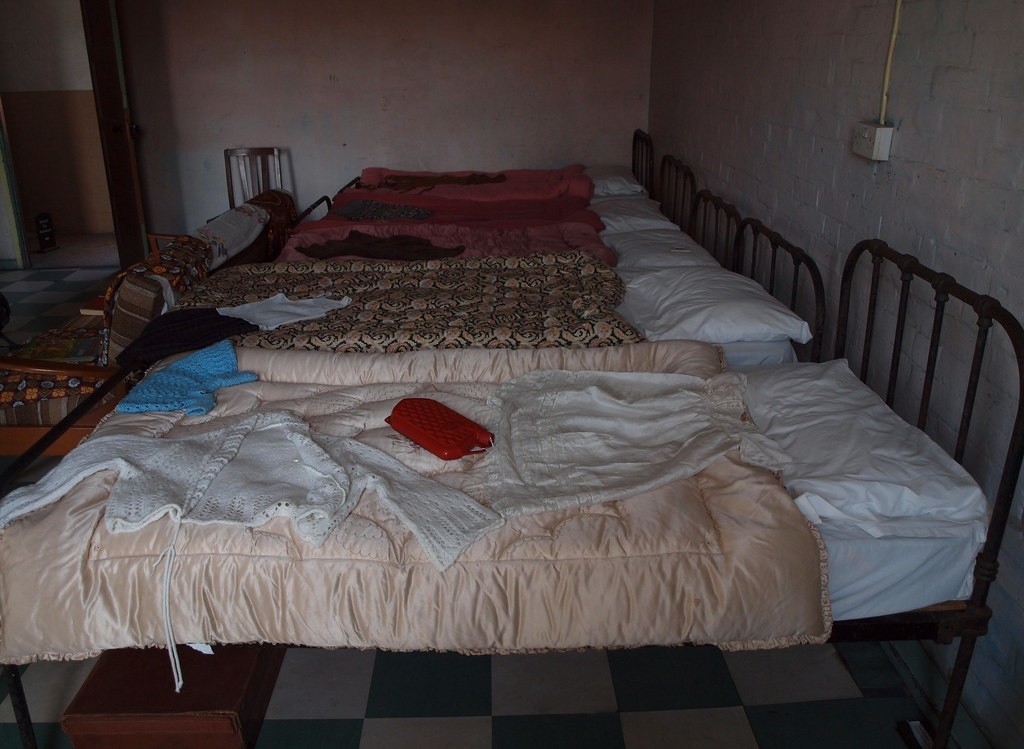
[{"xmin": 0, "ymin": 191, "xmax": 297, "ymax": 457}]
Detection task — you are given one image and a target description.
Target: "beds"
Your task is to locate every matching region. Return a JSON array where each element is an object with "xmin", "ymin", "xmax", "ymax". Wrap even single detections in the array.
[{"xmin": 0, "ymin": 129, "xmax": 1024, "ymax": 749}]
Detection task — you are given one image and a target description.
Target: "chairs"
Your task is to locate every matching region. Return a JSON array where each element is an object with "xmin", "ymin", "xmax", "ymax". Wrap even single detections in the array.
[{"xmin": 207, "ymin": 147, "xmax": 282, "ymax": 222}]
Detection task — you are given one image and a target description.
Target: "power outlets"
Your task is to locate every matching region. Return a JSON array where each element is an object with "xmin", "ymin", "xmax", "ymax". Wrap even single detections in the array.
[{"xmin": 852, "ymin": 121, "xmax": 894, "ymax": 161}]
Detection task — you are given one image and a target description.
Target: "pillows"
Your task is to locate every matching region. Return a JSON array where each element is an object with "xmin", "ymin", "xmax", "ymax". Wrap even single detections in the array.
[
  {"xmin": 603, "ymin": 229, "xmax": 715, "ymax": 268},
  {"xmin": 587, "ymin": 198, "xmax": 680, "ymax": 234},
  {"xmin": 584, "ymin": 165, "xmax": 643, "ymax": 195},
  {"xmin": 627, "ymin": 268, "xmax": 814, "ymax": 344},
  {"xmin": 745, "ymin": 358, "xmax": 987, "ymax": 537}
]
